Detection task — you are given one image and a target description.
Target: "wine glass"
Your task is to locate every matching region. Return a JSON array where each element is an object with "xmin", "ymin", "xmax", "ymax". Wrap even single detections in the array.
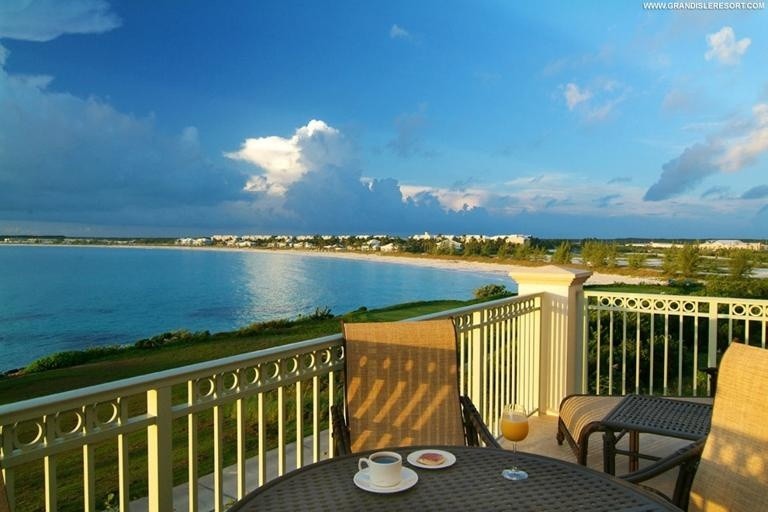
[{"xmin": 501, "ymin": 404, "xmax": 529, "ymax": 481}]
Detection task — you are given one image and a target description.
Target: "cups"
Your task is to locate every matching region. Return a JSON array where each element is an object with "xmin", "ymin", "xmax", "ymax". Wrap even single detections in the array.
[{"xmin": 358, "ymin": 451, "xmax": 403, "ymax": 487}]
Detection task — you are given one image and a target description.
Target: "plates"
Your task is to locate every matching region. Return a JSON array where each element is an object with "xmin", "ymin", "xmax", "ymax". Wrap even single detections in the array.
[
  {"xmin": 354, "ymin": 464, "xmax": 419, "ymax": 495},
  {"xmin": 407, "ymin": 449, "xmax": 456, "ymax": 469}
]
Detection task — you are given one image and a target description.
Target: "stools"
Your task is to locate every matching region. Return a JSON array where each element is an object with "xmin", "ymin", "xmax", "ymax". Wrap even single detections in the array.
[{"xmin": 598, "ymin": 393, "xmax": 714, "ymax": 508}]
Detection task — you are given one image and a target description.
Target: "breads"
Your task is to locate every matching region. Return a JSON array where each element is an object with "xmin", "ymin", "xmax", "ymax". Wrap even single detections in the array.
[{"xmin": 417, "ymin": 453, "xmax": 444, "ymax": 465}]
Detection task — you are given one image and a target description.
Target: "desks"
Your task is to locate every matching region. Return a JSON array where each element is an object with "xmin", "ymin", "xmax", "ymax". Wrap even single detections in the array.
[{"xmin": 223, "ymin": 443, "xmax": 685, "ymax": 510}]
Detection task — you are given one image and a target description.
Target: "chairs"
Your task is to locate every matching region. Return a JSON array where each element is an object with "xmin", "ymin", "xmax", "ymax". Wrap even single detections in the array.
[
  {"xmin": 329, "ymin": 318, "xmax": 503, "ymax": 458},
  {"xmin": 550, "ymin": 392, "xmax": 715, "ymax": 467},
  {"xmin": 670, "ymin": 334, "xmax": 768, "ymax": 511}
]
